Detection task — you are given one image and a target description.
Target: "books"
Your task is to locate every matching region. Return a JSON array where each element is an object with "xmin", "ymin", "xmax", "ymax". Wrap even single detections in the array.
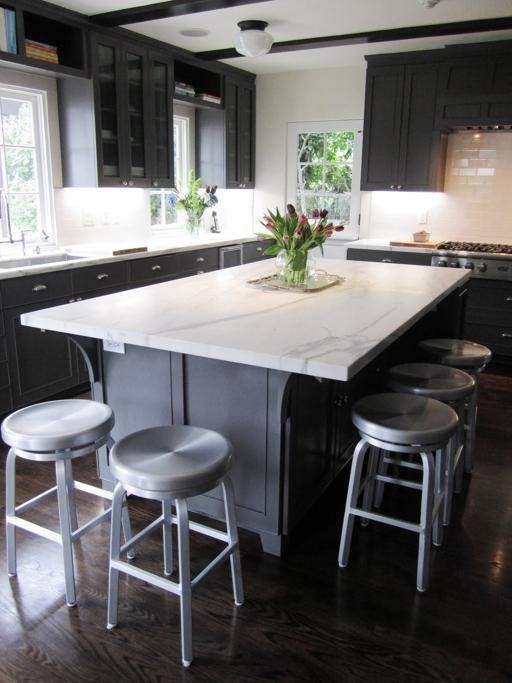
[
  {"xmin": 101, "ymin": 128, "xmax": 145, "ymax": 176},
  {"xmin": 1, "ymin": 6, "xmax": 59, "ymax": 64},
  {"xmin": 174, "ymin": 79, "xmax": 222, "ymax": 103}
]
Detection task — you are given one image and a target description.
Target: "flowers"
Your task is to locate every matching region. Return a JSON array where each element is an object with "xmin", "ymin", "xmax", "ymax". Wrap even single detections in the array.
[
  {"xmin": 167, "ymin": 167, "xmax": 219, "ymax": 217},
  {"xmin": 253, "ymin": 201, "xmax": 345, "ymax": 271}
]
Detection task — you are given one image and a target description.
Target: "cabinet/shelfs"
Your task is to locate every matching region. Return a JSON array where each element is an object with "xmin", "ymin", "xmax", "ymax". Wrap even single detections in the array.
[
  {"xmin": 4, "ymin": 258, "xmax": 132, "ymax": 410},
  {"xmin": 194, "ymin": 72, "xmax": 257, "ymax": 190},
  {"xmin": 131, "ymin": 247, "xmax": 219, "ymax": 288},
  {"xmin": 457, "ymin": 277, "xmax": 511, "ymax": 377},
  {"xmin": 359, "ymin": 47, "xmax": 449, "ymax": 193},
  {"xmin": 55, "ymin": 27, "xmax": 149, "ymax": 188},
  {"xmin": 147, "ymin": 43, "xmax": 176, "ymax": 188}
]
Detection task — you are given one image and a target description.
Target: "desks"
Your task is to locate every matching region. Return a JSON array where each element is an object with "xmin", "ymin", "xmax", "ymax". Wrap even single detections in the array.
[{"xmin": 20, "ymin": 254, "xmax": 474, "ymax": 559}]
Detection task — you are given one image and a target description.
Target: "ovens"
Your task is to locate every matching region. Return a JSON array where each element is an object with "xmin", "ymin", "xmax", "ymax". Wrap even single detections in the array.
[{"xmin": 218, "ymin": 244, "xmax": 244, "ymax": 270}]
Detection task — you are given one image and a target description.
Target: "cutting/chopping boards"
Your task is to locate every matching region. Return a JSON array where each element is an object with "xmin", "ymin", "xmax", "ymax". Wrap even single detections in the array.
[{"xmin": 389, "ymin": 238, "xmax": 446, "ymax": 249}]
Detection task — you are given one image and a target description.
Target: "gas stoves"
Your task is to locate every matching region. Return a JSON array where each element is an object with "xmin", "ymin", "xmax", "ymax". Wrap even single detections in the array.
[{"xmin": 431, "ymin": 241, "xmax": 512, "ymax": 281}]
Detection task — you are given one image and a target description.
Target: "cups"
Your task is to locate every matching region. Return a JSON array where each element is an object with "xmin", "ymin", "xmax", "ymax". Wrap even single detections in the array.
[{"xmin": 307, "ymin": 268, "xmax": 329, "ymax": 288}]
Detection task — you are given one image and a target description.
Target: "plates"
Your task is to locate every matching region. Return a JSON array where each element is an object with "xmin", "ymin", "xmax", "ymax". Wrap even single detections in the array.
[{"xmin": 103, "ymin": 164, "xmax": 145, "ymax": 177}]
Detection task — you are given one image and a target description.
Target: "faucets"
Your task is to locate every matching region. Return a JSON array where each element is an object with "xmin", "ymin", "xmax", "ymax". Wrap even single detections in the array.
[{"xmin": 20, "ymin": 229, "xmax": 31, "ymax": 256}]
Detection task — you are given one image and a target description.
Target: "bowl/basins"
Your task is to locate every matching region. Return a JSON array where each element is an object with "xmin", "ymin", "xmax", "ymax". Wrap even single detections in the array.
[
  {"xmin": 102, "ymin": 129, "xmax": 113, "ymax": 139},
  {"xmin": 413, "ymin": 231, "xmax": 431, "ymax": 243}
]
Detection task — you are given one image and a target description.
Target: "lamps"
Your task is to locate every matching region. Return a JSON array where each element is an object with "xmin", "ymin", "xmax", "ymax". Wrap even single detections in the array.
[{"xmin": 234, "ymin": 18, "xmax": 275, "ymax": 57}]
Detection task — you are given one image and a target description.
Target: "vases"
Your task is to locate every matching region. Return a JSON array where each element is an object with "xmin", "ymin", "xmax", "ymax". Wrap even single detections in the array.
[
  {"xmin": 276, "ymin": 247, "xmax": 313, "ymax": 288},
  {"xmin": 186, "ymin": 216, "xmax": 202, "ymax": 234}
]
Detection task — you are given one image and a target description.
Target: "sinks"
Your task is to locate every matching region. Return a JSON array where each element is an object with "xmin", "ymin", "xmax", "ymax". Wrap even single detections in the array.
[{"xmin": 0, "ymin": 253, "xmax": 88, "ymax": 270}]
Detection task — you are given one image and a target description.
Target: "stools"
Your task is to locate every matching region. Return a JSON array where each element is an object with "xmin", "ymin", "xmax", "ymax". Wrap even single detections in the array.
[
  {"xmin": 1, "ymin": 396, "xmax": 139, "ymax": 610},
  {"xmin": 373, "ymin": 361, "xmax": 476, "ymax": 526},
  {"xmin": 417, "ymin": 333, "xmax": 494, "ymax": 477},
  {"xmin": 335, "ymin": 390, "xmax": 462, "ymax": 594},
  {"xmin": 106, "ymin": 423, "xmax": 247, "ymax": 671}
]
